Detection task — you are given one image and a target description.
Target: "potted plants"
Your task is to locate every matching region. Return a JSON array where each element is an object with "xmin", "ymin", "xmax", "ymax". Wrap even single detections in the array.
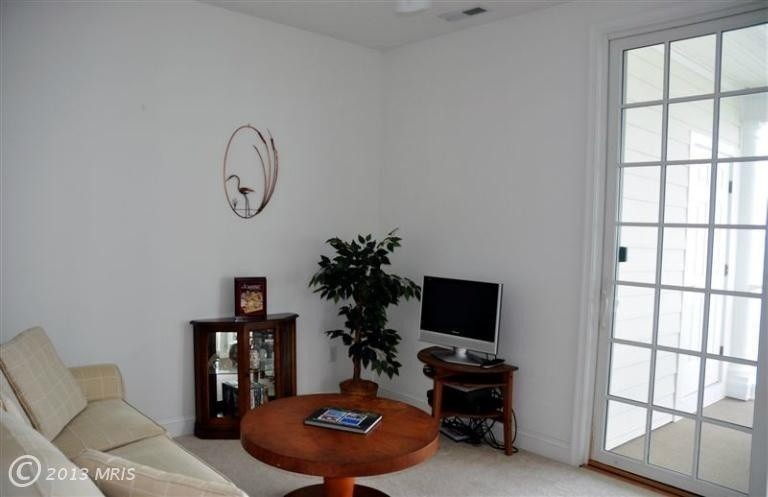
[{"xmin": 306, "ymin": 226, "xmax": 421, "ymax": 398}]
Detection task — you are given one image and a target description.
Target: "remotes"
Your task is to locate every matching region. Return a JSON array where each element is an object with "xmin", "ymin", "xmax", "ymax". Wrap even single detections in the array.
[{"xmin": 482, "ymin": 358, "xmax": 505, "ymax": 369}]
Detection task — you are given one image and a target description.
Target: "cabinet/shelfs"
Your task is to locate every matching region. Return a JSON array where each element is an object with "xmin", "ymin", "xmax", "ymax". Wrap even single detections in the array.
[
  {"xmin": 189, "ymin": 312, "xmax": 300, "ymax": 443},
  {"xmin": 416, "ymin": 345, "xmax": 519, "ymax": 456}
]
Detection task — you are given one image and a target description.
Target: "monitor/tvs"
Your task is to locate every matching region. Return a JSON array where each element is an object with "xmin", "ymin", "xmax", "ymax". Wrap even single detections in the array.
[{"xmin": 418, "ymin": 274, "xmax": 503, "ymax": 367}]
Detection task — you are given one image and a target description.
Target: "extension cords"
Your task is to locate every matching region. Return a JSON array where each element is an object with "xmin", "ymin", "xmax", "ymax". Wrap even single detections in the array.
[{"xmin": 439, "ymin": 425, "xmax": 469, "ymax": 442}]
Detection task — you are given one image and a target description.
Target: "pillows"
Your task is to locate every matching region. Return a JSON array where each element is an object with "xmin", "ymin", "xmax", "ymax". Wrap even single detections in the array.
[
  {"xmin": 1, "ymin": 326, "xmax": 89, "ymax": 444},
  {"xmin": 70, "ymin": 446, "xmax": 249, "ymax": 497}
]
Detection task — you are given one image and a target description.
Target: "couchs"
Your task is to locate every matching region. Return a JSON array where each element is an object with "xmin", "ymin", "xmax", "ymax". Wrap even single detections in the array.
[{"xmin": 0, "ymin": 361, "xmax": 250, "ymax": 497}]
